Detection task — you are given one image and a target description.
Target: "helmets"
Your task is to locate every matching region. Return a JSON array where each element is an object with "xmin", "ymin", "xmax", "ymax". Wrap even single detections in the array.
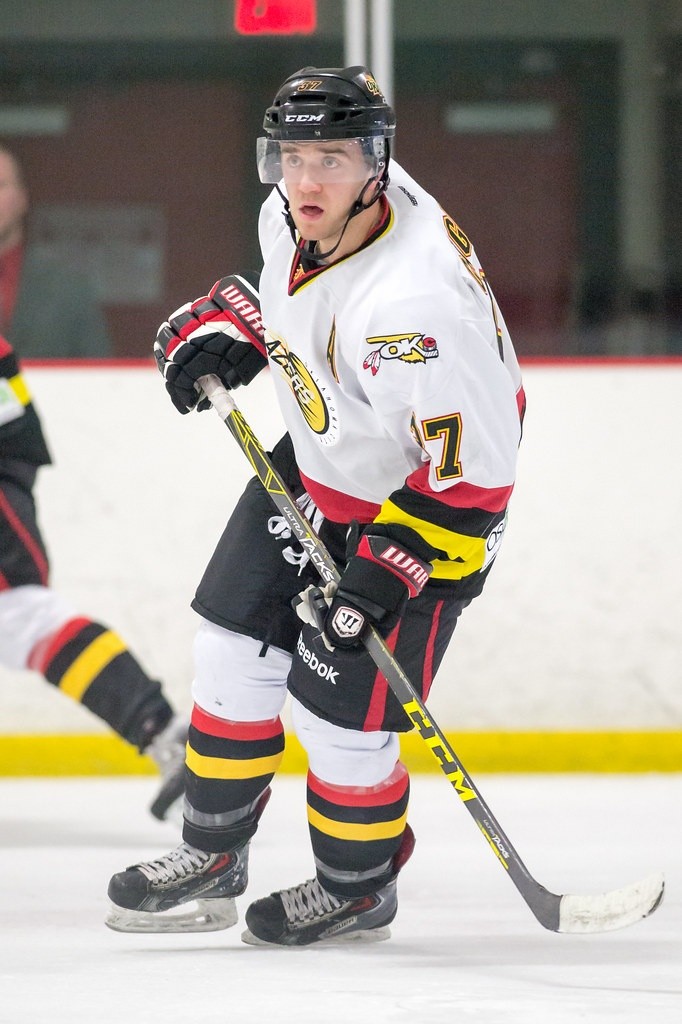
[{"xmin": 260, "ymin": 67, "xmax": 396, "ymax": 142}]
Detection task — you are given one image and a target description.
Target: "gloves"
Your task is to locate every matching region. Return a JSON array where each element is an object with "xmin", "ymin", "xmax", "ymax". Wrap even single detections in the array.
[
  {"xmin": 153, "ymin": 274, "xmax": 267, "ymax": 415},
  {"xmin": 291, "ymin": 521, "xmax": 434, "ymax": 660}
]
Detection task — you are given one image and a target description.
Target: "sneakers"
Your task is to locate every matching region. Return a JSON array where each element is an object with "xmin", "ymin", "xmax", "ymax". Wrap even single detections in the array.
[
  {"xmin": 104, "ymin": 786, "xmax": 271, "ymax": 934},
  {"xmin": 242, "ymin": 822, "xmax": 416, "ymax": 951}
]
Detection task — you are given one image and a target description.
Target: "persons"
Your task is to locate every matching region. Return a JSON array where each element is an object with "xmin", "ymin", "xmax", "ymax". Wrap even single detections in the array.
[
  {"xmin": 107, "ymin": 66, "xmax": 526, "ymax": 951},
  {"xmin": 0, "ymin": 140, "xmax": 114, "ymax": 358},
  {"xmin": 0, "ymin": 338, "xmax": 188, "ymax": 823}
]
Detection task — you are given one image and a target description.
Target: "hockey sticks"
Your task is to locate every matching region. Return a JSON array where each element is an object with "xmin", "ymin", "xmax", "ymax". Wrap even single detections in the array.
[{"xmin": 195, "ymin": 366, "xmax": 667, "ymax": 937}]
